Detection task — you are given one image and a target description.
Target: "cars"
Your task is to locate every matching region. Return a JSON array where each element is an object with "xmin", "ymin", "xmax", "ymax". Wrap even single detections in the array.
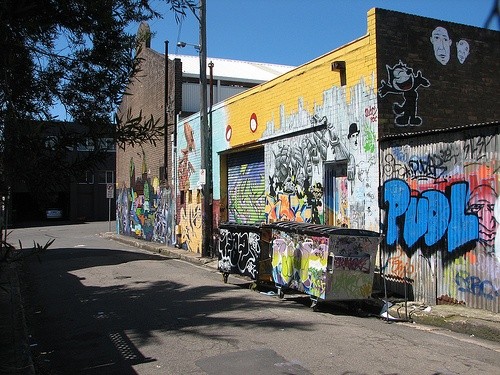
[{"xmin": 46, "ymin": 208, "xmax": 63, "ymax": 219}]
